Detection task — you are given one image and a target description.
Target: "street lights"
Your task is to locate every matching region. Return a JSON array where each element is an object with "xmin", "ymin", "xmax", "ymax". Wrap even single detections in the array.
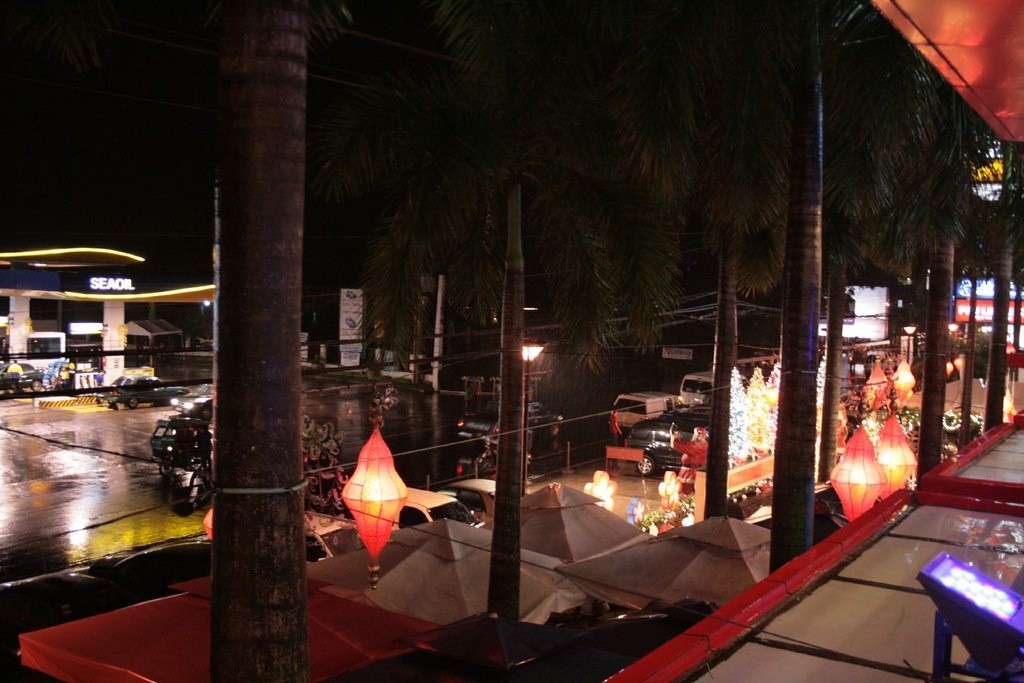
[
  {"xmin": 902, "ymin": 322, "xmax": 920, "ymax": 364},
  {"xmin": 949, "ymin": 321, "xmax": 960, "ymax": 361}
]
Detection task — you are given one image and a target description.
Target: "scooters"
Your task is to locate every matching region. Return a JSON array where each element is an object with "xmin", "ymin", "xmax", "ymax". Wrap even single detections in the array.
[
  {"xmin": 159, "ymin": 436, "xmax": 205, "ymax": 477},
  {"xmin": 150, "ymin": 419, "xmax": 210, "ymax": 464}
]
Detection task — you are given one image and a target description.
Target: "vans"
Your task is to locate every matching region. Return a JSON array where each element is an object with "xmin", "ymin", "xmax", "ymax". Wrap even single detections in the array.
[{"xmin": 608, "ymin": 369, "xmax": 748, "ymax": 478}]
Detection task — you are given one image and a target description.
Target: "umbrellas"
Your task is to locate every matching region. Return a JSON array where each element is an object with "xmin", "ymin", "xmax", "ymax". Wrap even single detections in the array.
[{"xmin": 304, "ymin": 481, "xmax": 770, "ymax": 631}]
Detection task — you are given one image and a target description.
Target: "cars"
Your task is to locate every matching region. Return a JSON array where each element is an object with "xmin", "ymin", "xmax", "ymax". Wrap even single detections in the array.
[
  {"xmin": 456, "ymin": 401, "xmax": 579, "ymax": 436},
  {"xmin": 169, "ymin": 383, "xmax": 214, "ymax": 419},
  {"xmin": 0, "ymin": 478, "xmax": 496, "ymax": 649},
  {"xmin": 101, "ymin": 374, "xmax": 190, "ymax": 410},
  {"xmin": 0, "ymin": 361, "xmax": 44, "ymax": 392}
]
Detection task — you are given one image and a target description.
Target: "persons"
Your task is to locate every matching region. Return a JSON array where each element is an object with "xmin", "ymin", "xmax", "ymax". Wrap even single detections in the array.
[
  {"xmin": 61, "ymin": 367, "xmax": 70, "ymax": 380},
  {"xmin": 608, "ymin": 410, "xmax": 622, "ymax": 446},
  {"xmin": 95, "ymin": 370, "xmax": 106, "ymax": 386},
  {"xmin": 6, "ymin": 360, "xmax": 23, "ymax": 373}
]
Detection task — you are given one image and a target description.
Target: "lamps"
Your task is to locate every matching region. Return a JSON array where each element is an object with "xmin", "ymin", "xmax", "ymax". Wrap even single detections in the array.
[
  {"xmin": 914, "ymin": 550, "xmax": 1024, "ymax": 672},
  {"xmin": 944, "ymin": 355, "xmax": 960, "ymax": 378},
  {"xmin": 828, "ymin": 349, "xmax": 917, "ymax": 522},
  {"xmin": 339, "ymin": 383, "xmax": 408, "ymax": 590}
]
